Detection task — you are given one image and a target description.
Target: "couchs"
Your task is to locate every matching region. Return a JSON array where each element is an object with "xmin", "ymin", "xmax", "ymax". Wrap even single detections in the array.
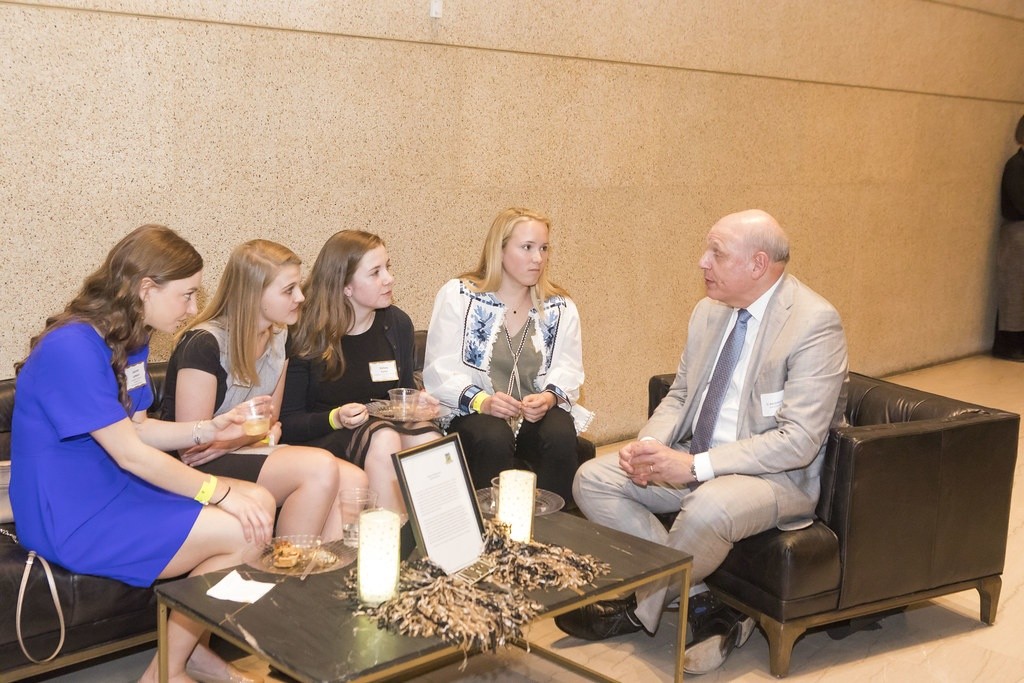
[
  {"xmin": 646, "ymin": 373, "xmax": 1020, "ymax": 678},
  {"xmin": 0, "ymin": 330, "xmax": 429, "ymax": 683}
]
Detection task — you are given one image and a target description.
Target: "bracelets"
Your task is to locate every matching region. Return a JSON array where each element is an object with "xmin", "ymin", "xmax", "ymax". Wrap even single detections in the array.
[
  {"xmin": 472, "ymin": 392, "xmax": 490, "ymax": 415},
  {"xmin": 195, "ymin": 472, "xmax": 232, "ymax": 508},
  {"xmin": 192, "ymin": 419, "xmax": 208, "ymax": 446},
  {"xmin": 690, "ymin": 454, "xmax": 700, "ymax": 485}
]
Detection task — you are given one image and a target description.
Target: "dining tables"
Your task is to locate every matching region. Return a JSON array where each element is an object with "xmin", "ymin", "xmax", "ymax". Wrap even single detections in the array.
[{"xmin": 155, "ymin": 512, "xmax": 694, "ymax": 683}]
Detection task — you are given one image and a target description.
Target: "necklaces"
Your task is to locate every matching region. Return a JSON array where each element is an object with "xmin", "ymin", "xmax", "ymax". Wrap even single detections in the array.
[{"xmin": 497, "ymin": 288, "xmax": 520, "ymax": 314}]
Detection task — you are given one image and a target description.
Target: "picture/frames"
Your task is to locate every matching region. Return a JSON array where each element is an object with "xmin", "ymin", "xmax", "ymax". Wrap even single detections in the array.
[{"xmin": 391, "ymin": 432, "xmax": 487, "ymax": 576}]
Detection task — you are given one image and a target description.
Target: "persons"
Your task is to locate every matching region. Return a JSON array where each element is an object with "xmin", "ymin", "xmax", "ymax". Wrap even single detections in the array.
[
  {"xmin": 9, "ymin": 222, "xmax": 264, "ymax": 683},
  {"xmin": 551, "ymin": 208, "xmax": 849, "ymax": 675},
  {"xmin": 988, "ymin": 116, "xmax": 1024, "ymax": 361},
  {"xmin": 158, "ymin": 238, "xmax": 369, "ymax": 559},
  {"xmin": 278, "ymin": 230, "xmax": 444, "ymax": 532},
  {"xmin": 424, "ymin": 205, "xmax": 584, "ymax": 518}
]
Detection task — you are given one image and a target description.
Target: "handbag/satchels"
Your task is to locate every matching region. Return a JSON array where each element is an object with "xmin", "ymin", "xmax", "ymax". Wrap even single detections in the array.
[{"xmin": 0, "ymin": 460, "xmax": 15, "ymax": 524}]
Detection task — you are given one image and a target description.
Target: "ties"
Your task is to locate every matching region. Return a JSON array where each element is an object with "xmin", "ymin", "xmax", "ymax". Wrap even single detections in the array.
[{"xmin": 688, "ymin": 309, "xmax": 752, "ymax": 492}]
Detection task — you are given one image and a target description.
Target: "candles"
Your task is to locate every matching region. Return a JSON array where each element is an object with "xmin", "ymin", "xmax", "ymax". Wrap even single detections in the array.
[
  {"xmin": 358, "ymin": 508, "xmax": 401, "ymax": 609},
  {"xmin": 498, "ymin": 469, "xmax": 536, "ymax": 542}
]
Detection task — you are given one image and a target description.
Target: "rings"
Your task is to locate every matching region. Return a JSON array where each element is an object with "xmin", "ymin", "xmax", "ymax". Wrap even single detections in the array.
[{"xmin": 649, "ymin": 465, "xmax": 653, "ymax": 472}]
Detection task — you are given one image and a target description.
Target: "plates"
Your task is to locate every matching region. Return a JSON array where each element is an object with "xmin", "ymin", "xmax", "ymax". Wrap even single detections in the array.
[
  {"xmin": 474, "ymin": 487, "xmax": 566, "ymax": 515},
  {"xmin": 364, "ymin": 400, "xmax": 452, "ymax": 421},
  {"xmin": 242, "ymin": 535, "xmax": 357, "ymax": 577}
]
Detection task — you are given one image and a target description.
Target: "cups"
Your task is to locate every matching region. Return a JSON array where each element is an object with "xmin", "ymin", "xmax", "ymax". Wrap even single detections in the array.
[
  {"xmin": 388, "ymin": 388, "xmax": 421, "ymax": 419},
  {"xmin": 491, "ymin": 475, "xmax": 501, "ymax": 514},
  {"xmin": 337, "ymin": 489, "xmax": 378, "ymax": 547},
  {"xmin": 238, "ymin": 399, "xmax": 270, "ymax": 436}
]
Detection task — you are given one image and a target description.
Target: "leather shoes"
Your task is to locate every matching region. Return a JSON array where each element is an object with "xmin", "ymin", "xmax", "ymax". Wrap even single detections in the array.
[
  {"xmin": 683, "ymin": 603, "xmax": 756, "ymax": 675},
  {"xmin": 554, "ymin": 598, "xmax": 641, "ymax": 641},
  {"xmin": 188, "ymin": 661, "xmax": 254, "ymax": 683}
]
